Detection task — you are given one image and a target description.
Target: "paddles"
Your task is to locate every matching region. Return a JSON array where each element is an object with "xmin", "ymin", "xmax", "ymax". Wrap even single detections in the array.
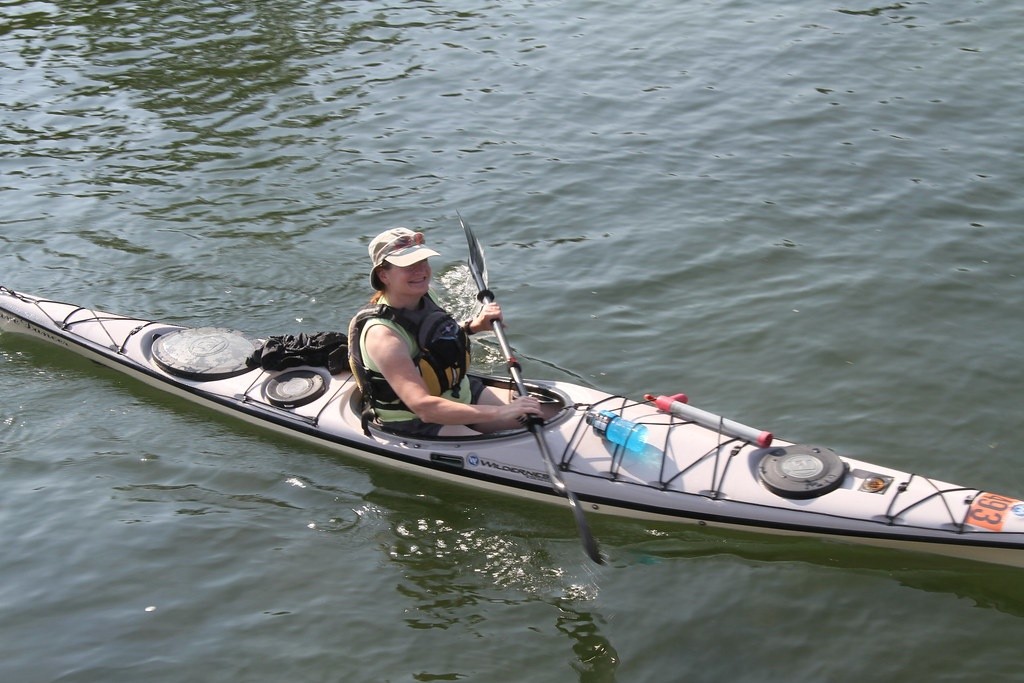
[{"xmin": 453, "ymin": 204, "xmax": 607, "ymax": 567}]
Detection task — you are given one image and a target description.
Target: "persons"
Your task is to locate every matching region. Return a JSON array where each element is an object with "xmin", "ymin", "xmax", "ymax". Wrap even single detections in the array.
[{"xmin": 347, "ymin": 226, "xmax": 561, "ymax": 438}]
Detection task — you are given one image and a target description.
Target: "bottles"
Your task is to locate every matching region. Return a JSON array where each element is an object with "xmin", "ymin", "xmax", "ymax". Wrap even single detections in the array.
[{"xmin": 586, "ymin": 410, "xmax": 648, "ymax": 453}]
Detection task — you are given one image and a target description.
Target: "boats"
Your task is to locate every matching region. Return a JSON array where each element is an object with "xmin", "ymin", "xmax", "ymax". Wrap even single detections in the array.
[{"xmin": 0, "ymin": 283, "xmax": 1024, "ymax": 576}]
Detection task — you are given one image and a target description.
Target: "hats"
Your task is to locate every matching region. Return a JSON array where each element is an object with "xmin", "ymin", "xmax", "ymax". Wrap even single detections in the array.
[{"xmin": 368, "ymin": 228, "xmax": 441, "ymax": 291}]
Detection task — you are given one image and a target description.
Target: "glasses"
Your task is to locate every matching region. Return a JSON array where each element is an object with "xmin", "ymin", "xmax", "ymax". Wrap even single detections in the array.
[{"xmin": 375, "ymin": 232, "xmax": 426, "ymax": 264}]
